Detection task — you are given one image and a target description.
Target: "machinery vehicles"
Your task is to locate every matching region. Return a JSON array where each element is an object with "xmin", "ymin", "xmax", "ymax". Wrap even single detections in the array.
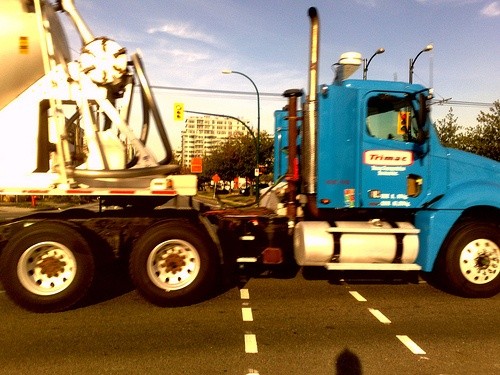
[{"xmin": 1, "ymin": 1, "xmax": 500, "ymax": 314}]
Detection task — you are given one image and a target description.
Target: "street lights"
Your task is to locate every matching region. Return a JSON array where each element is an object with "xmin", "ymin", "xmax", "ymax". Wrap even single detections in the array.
[
  {"xmin": 221, "ymin": 70, "xmax": 254, "ymax": 202},
  {"xmin": 409, "ymin": 45, "xmax": 433, "ymax": 84},
  {"xmin": 363, "ymin": 48, "xmax": 385, "ymax": 80}
]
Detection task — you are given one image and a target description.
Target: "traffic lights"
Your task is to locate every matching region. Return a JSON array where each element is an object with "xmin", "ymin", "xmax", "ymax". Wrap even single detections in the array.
[{"xmin": 173, "ymin": 102, "xmax": 185, "ymax": 122}]
